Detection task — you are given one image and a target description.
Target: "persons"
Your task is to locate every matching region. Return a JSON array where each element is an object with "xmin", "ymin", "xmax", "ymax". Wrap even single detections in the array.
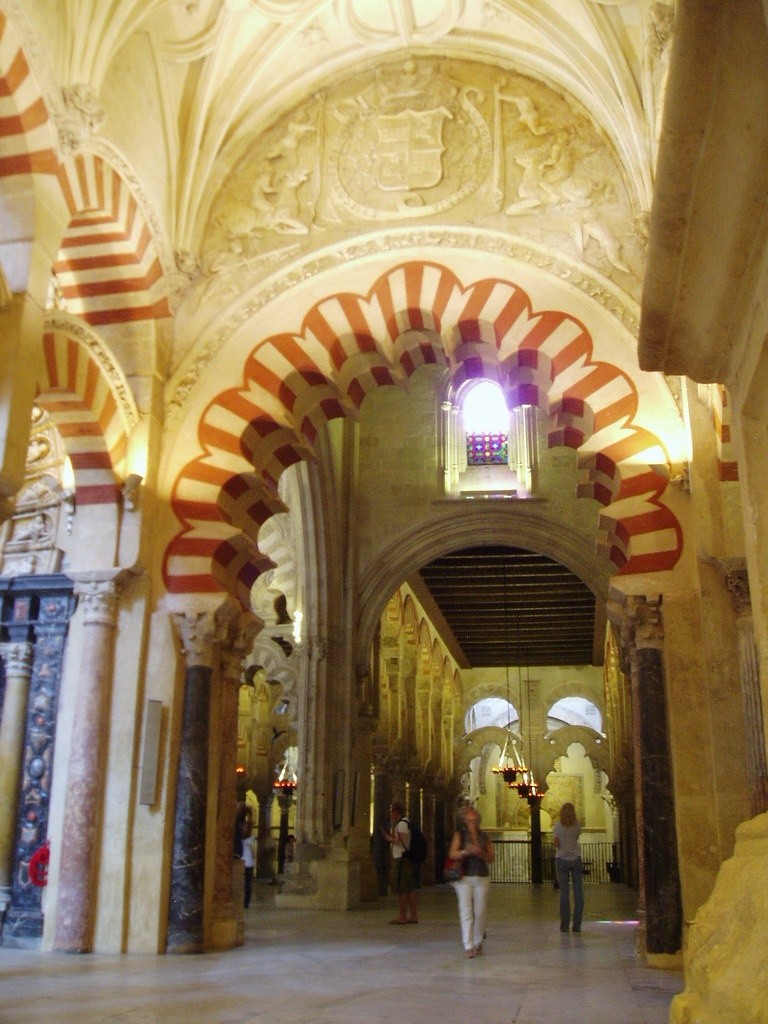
[
  {"xmin": 554, "ymin": 803, "xmax": 584, "ymax": 932},
  {"xmin": 448, "ymin": 807, "xmax": 493, "ymax": 957},
  {"xmin": 385, "ymin": 803, "xmax": 418, "ymax": 923},
  {"xmin": 241, "ymin": 822, "xmax": 254, "ymax": 908},
  {"xmin": 284, "ymin": 835, "xmax": 294, "ymax": 871}
]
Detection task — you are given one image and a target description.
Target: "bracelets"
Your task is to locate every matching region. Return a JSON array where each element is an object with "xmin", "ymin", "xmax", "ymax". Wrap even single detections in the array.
[{"xmin": 462, "ymin": 851, "xmax": 465, "ymax": 857}]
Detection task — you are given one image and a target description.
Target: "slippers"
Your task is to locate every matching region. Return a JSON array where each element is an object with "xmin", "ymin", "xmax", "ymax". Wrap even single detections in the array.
[
  {"xmin": 407, "ymin": 920, "xmax": 419, "ymax": 923},
  {"xmin": 388, "ymin": 919, "xmax": 407, "ymax": 925}
]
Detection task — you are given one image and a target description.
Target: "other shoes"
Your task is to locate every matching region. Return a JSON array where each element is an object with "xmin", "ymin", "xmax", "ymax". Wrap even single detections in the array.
[
  {"xmin": 474, "ymin": 945, "xmax": 482, "ymax": 955},
  {"xmin": 464, "ymin": 949, "xmax": 474, "ymax": 958}
]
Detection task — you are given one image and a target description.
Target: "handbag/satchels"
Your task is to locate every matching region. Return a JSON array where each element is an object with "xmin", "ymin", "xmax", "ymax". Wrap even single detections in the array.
[{"xmin": 444, "ymin": 831, "xmax": 462, "ymax": 880}]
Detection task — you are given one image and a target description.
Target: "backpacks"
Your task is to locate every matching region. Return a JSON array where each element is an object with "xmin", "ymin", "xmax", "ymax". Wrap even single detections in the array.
[{"xmin": 397, "ymin": 820, "xmax": 428, "ymax": 865}]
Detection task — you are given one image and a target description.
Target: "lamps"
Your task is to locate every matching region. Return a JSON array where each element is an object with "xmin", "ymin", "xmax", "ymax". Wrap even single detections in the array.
[
  {"xmin": 122, "ymin": 474, "xmax": 143, "ymax": 509},
  {"xmin": 491, "ymin": 556, "xmax": 544, "ymax": 808},
  {"xmin": 667, "ymin": 462, "xmax": 690, "ymax": 493}
]
